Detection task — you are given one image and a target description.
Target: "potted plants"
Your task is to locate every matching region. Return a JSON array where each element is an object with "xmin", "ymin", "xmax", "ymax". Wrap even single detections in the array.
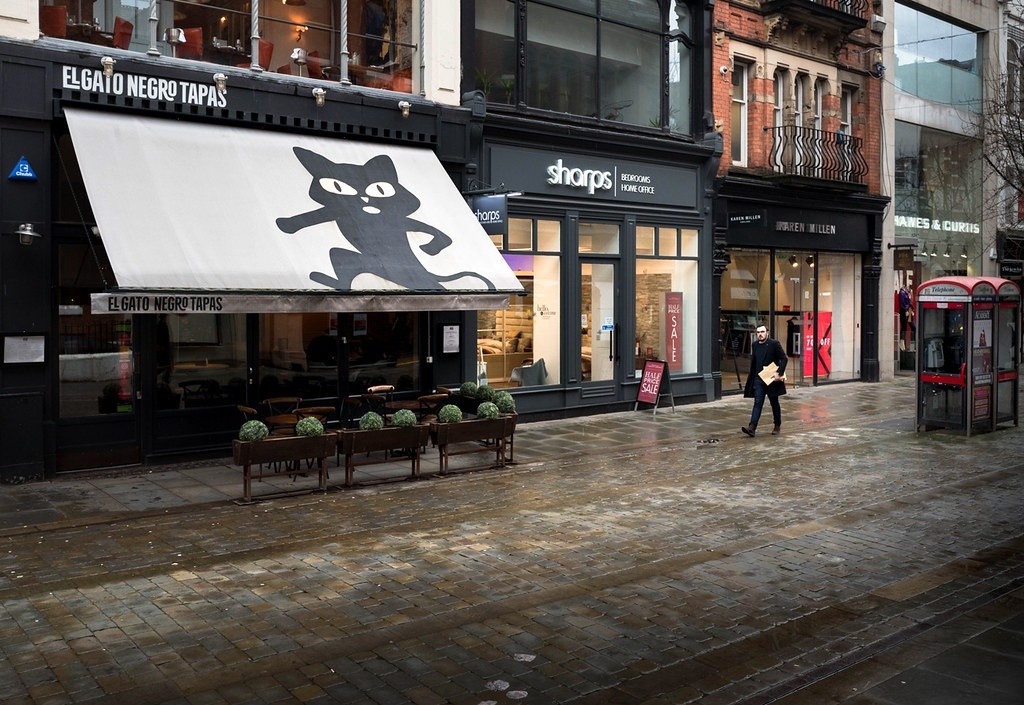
[
  {"xmin": 490, "ymin": 390, "xmax": 518, "ymax": 437},
  {"xmin": 338, "ymin": 409, "xmax": 432, "ymax": 487},
  {"xmin": 230, "ymin": 415, "xmax": 337, "ymax": 503},
  {"xmin": 476, "ymin": 68, "xmax": 516, "ymax": 103},
  {"xmin": 451, "ymin": 381, "xmax": 493, "ymax": 414},
  {"xmin": 430, "ymin": 402, "xmax": 511, "ymax": 476}
]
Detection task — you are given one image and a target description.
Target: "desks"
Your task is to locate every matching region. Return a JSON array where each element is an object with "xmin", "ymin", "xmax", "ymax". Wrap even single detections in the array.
[
  {"xmin": 265, "ymin": 412, "xmax": 322, "ymax": 435},
  {"xmin": 384, "ymin": 398, "xmax": 437, "ymax": 420}
]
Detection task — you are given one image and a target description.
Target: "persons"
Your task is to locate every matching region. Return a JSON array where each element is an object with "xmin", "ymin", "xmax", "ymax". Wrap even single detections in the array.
[
  {"xmin": 742, "ymin": 323, "xmax": 788, "ymax": 438},
  {"xmin": 899, "ymin": 282, "xmax": 917, "ymax": 350}
]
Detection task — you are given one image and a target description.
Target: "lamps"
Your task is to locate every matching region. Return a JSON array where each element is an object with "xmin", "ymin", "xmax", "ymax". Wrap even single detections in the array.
[
  {"xmin": 931, "ymin": 246, "xmax": 937, "ymax": 256},
  {"xmin": 806, "ymin": 257, "xmax": 814, "ymax": 268},
  {"xmin": 788, "ymin": 256, "xmax": 797, "ymax": 267},
  {"xmin": 961, "ymin": 249, "xmax": 967, "ymax": 258},
  {"xmin": 943, "ymin": 247, "xmax": 951, "ymax": 257},
  {"xmin": 921, "ymin": 246, "xmax": 928, "ymax": 256}
]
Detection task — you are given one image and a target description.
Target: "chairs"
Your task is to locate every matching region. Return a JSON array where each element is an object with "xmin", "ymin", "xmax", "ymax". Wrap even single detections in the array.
[
  {"xmin": 364, "ymin": 383, "xmax": 396, "ymax": 413},
  {"xmin": 237, "ymin": 404, "xmax": 255, "ymax": 423},
  {"xmin": 292, "ymin": 406, "xmax": 332, "ymax": 480},
  {"xmin": 262, "ymin": 396, "xmax": 302, "ymax": 414},
  {"xmin": 437, "ymin": 384, "xmax": 455, "ymax": 405},
  {"xmin": 417, "ymin": 392, "xmax": 447, "ymax": 420},
  {"xmin": 40, "ymin": 2, "xmax": 411, "ymax": 93}
]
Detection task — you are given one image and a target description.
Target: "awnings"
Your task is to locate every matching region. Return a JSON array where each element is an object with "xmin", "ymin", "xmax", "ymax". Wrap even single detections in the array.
[{"xmin": 60, "ymin": 105, "xmax": 527, "ymax": 315}]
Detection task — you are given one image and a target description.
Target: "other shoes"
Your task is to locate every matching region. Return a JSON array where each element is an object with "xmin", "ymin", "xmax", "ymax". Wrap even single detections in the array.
[
  {"xmin": 742, "ymin": 425, "xmax": 755, "ymax": 437},
  {"xmin": 772, "ymin": 426, "xmax": 780, "ymax": 435}
]
